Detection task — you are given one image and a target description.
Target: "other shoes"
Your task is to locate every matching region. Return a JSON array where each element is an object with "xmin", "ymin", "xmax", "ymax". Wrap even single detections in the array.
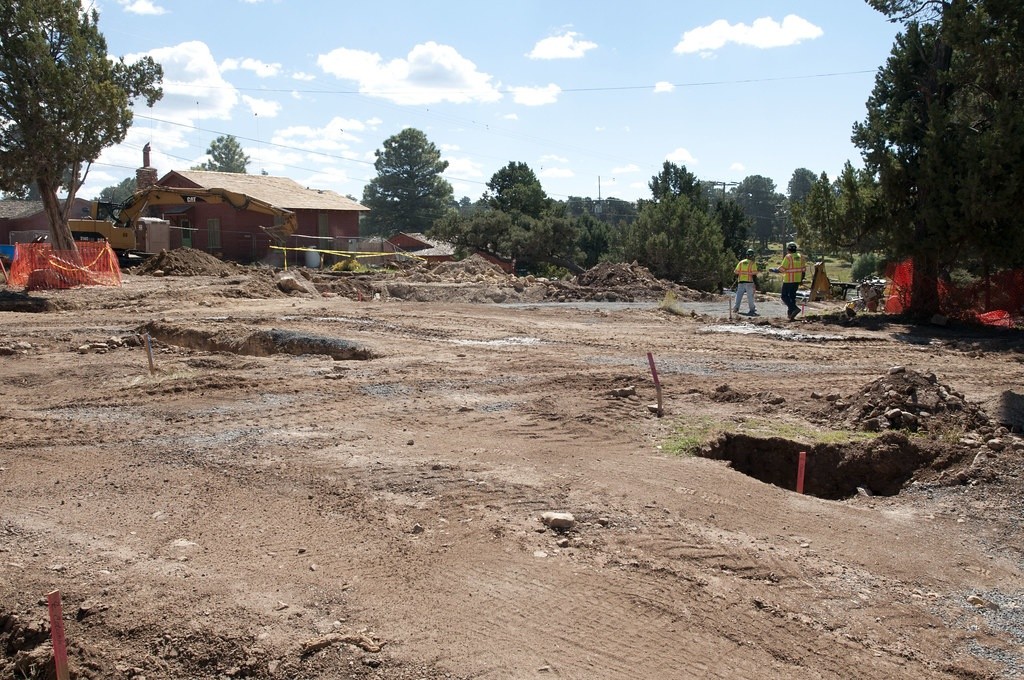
[
  {"xmin": 733, "ymin": 308, "xmax": 738, "ymax": 313},
  {"xmin": 748, "ymin": 312, "xmax": 760, "ymax": 317},
  {"xmin": 790, "ymin": 308, "xmax": 801, "ymax": 318},
  {"xmin": 788, "ymin": 315, "xmax": 794, "ymax": 320}
]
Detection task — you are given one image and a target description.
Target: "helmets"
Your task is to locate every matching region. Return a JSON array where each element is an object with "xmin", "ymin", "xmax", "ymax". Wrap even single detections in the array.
[
  {"xmin": 787, "ymin": 242, "xmax": 797, "ymax": 250},
  {"xmin": 746, "ymin": 249, "xmax": 754, "ymax": 257}
]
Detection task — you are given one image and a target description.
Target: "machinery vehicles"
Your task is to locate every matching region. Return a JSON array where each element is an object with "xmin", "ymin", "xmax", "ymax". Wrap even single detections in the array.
[{"xmin": 65, "ymin": 179, "xmax": 300, "ymax": 267}]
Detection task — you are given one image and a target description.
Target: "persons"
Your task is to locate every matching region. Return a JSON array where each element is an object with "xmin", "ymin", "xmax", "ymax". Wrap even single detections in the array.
[
  {"xmin": 769, "ymin": 242, "xmax": 807, "ymax": 321},
  {"xmin": 733, "ymin": 249, "xmax": 761, "ymax": 316}
]
